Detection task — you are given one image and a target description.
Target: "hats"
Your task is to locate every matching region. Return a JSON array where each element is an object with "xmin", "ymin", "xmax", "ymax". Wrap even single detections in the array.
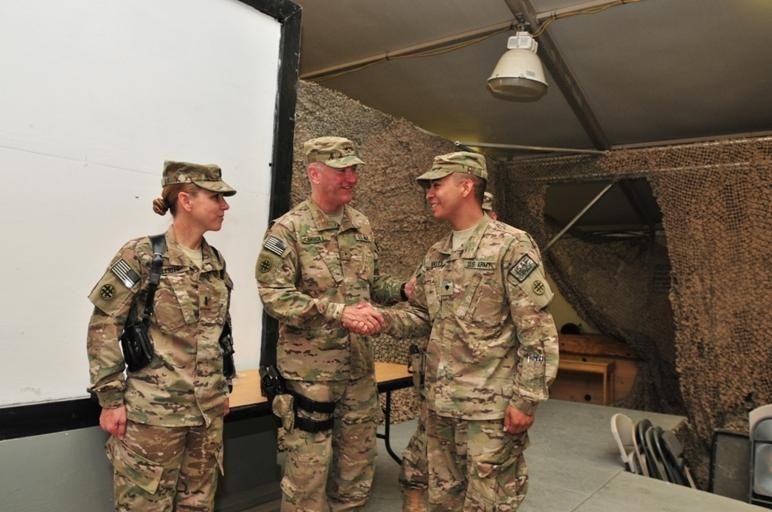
[
  {"xmin": 416, "ymin": 151, "xmax": 489, "ymax": 186},
  {"xmin": 162, "ymin": 161, "xmax": 237, "ymax": 198},
  {"xmin": 300, "ymin": 135, "xmax": 366, "ymax": 171}
]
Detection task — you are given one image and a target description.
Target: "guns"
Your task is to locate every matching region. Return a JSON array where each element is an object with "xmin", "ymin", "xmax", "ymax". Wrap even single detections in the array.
[
  {"xmin": 264, "ymin": 378, "xmax": 286, "ymax": 403},
  {"xmin": 410, "ymin": 344, "xmax": 419, "ymax": 354}
]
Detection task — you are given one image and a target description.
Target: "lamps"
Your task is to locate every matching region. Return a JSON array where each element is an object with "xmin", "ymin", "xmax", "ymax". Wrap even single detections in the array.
[{"xmin": 484, "ymin": 19, "xmax": 549, "ymax": 103}]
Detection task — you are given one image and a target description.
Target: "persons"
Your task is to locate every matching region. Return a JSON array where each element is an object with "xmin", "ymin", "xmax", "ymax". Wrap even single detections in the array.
[
  {"xmin": 254, "ymin": 133, "xmax": 416, "ymax": 511},
  {"xmin": 343, "ymin": 152, "xmax": 560, "ymax": 511},
  {"xmin": 86, "ymin": 161, "xmax": 239, "ymax": 512},
  {"xmin": 395, "ymin": 186, "xmax": 498, "ymax": 510}
]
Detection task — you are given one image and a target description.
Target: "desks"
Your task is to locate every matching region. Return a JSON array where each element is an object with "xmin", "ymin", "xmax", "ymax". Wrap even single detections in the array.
[{"xmin": 227, "ymin": 359, "xmax": 414, "ymax": 466}]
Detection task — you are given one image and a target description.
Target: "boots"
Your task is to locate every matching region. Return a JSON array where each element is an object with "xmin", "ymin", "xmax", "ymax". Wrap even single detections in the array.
[{"xmin": 398, "ymin": 486, "xmax": 426, "ymax": 511}]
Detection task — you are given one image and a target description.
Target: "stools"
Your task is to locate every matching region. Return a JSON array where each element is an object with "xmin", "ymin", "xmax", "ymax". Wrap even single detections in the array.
[{"xmin": 554, "ymin": 355, "xmax": 618, "ymax": 406}]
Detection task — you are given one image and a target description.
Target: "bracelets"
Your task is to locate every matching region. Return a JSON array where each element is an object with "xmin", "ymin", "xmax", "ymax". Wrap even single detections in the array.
[{"xmin": 400, "ymin": 283, "xmax": 410, "ymax": 302}]
